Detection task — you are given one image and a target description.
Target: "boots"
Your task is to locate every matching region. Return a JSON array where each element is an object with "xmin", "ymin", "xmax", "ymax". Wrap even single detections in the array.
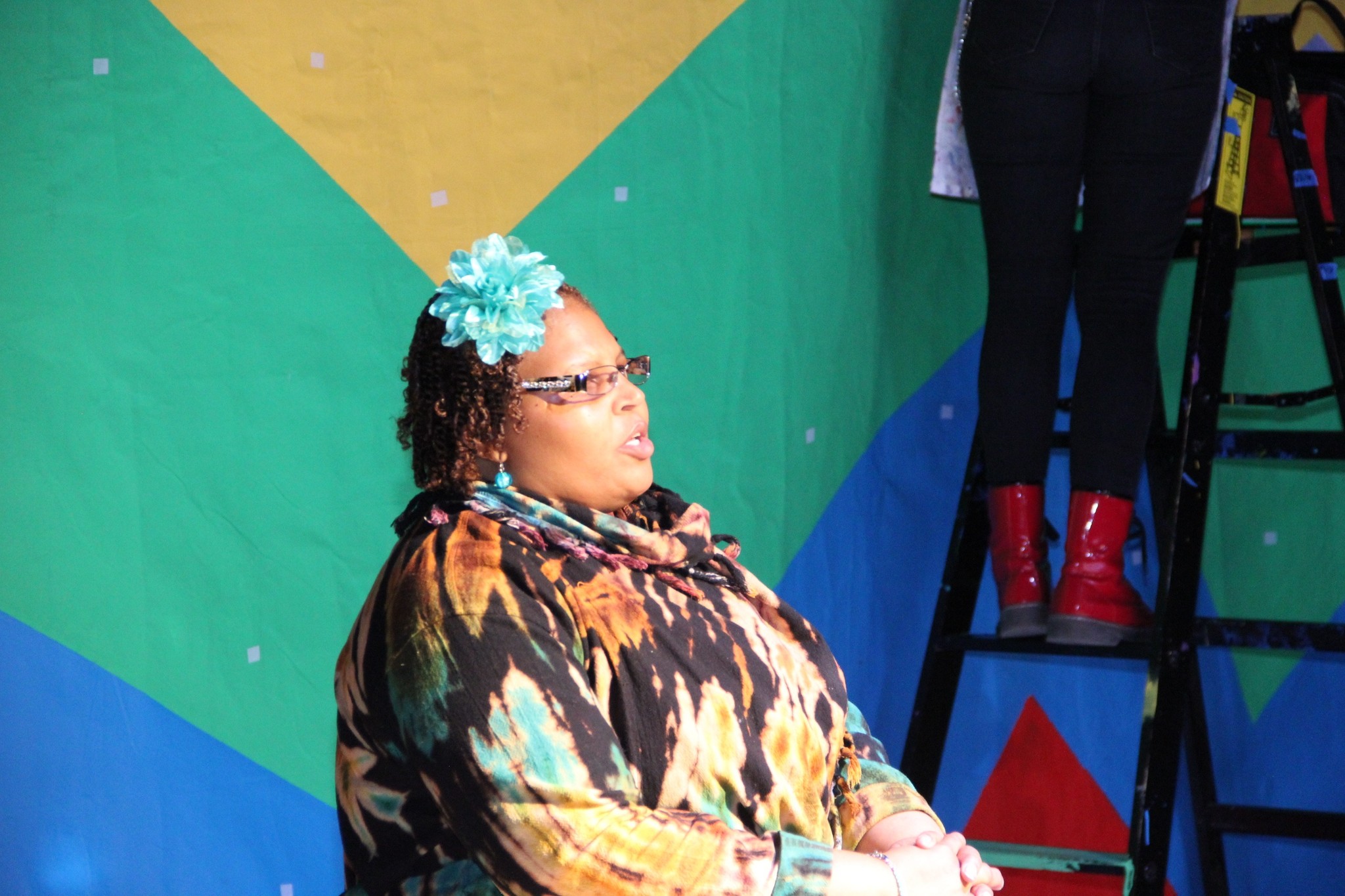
[
  {"xmin": 992, "ymin": 485, "xmax": 1050, "ymax": 637},
  {"xmin": 1048, "ymin": 492, "xmax": 1153, "ymax": 646}
]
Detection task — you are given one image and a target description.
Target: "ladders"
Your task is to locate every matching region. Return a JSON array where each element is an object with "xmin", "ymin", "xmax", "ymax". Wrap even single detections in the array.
[{"xmin": 898, "ymin": 13, "xmax": 1345, "ymax": 896}]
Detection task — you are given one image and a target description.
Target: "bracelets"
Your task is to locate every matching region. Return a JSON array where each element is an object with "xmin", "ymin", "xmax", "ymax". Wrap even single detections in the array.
[{"xmin": 869, "ymin": 851, "xmax": 902, "ymax": 896}]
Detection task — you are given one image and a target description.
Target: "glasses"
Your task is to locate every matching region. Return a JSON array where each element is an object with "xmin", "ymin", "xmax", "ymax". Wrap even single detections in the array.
[{"xmin": 521, "ymin": 355, "xmax": 651, "ymax": 396}]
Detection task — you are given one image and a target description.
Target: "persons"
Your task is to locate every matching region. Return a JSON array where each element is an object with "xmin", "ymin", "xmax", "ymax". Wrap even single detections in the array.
[
  {"xmin": 945, "ymin": 0, "xmax": 1225, "ymax": 648},
  {"xmin": 334, "ymin": 234, "xmax": 1008, "ymax": 896}
]
